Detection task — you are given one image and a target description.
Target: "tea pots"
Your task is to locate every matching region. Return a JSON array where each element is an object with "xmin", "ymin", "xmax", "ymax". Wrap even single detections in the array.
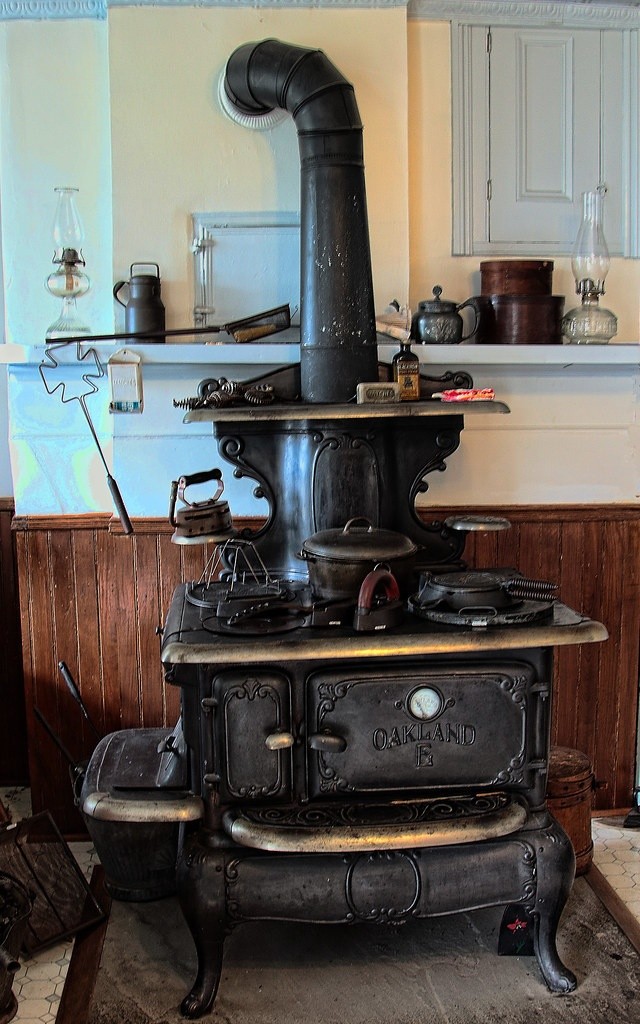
[{"xmin": 390, "ymin": 284, "xmax": 482, "ymax": 344}]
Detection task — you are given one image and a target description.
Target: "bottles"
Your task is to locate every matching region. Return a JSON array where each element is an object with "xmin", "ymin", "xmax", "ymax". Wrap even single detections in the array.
[{"xmin": 392, "ymin": 340, "xmax": 419, "ymax": 403}]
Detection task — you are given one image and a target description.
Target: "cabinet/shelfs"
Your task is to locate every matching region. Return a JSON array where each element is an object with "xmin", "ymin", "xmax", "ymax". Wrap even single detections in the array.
[{"xmin": 487, "ymin": 22, "xmax": 637, "ymax": 260}]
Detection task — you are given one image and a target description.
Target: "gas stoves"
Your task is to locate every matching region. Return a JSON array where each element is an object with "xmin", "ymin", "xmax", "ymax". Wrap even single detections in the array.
[{"xmin": 159, "ymin": 565, "xmax": 610, "ymax": 664}]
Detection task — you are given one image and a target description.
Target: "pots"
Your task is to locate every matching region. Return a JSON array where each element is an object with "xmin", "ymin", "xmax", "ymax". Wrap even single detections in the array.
[
  {"xmin": 293, "ymin": 515, "xmax": 424, "ymax": 603},
  {"xmin": 418, "ymin": 577, "xmax": 513, "ymax": 611}
]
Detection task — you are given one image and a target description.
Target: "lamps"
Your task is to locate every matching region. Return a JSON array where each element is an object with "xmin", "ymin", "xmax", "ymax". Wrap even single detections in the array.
[
  {"xmin": 44, "ymin": 181, "xmax": 94, "ymax": 339},
  {"xmin": 562, "ymin": 183, "xmax": 616, "ymax": 345}
]
